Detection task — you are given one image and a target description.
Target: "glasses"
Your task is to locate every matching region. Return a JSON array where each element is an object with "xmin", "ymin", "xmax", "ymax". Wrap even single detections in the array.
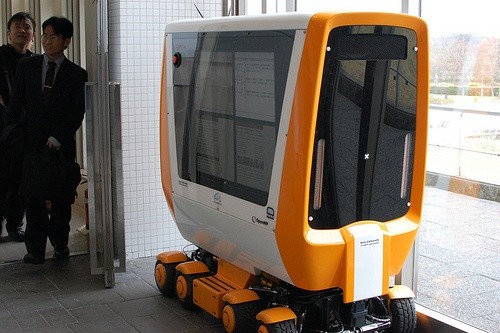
[{"xmin": 41, "ymin": 35, "xmax": 65, "ymax": 41}]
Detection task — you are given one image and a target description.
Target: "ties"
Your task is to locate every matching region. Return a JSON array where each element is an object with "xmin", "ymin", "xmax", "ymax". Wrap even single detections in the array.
[{"xmin": 43, "ymin": 59, "xmax": 57, "ymax": 93}]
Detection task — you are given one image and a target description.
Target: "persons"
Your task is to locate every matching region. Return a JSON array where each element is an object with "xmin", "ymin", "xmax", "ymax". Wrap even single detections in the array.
[
  {"xmin": 0, "ymin": 12, "xmax": 40, "ymax": 243},
  {"xmin": 5, "ymin": 17, "xmax": 88, "ymax": 263}
]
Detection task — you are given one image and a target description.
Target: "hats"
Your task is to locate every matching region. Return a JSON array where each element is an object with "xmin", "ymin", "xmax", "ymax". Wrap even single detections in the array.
[{"xmin": 24, "ymin": 143, "xmax": 63, "ymax": 184}]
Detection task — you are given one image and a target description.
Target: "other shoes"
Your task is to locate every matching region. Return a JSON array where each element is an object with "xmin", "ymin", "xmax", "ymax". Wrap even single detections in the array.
[
  {"xmin": 23, "ymin": 252, "xmax": 46, "ymax": 265},
  {"xmin": 7, "ymin": 227, "xmax": 25, "ymax": 242},
  {"xmin": 53, "ymin": 248, "xmax": 69, "ymax": 267}
]
